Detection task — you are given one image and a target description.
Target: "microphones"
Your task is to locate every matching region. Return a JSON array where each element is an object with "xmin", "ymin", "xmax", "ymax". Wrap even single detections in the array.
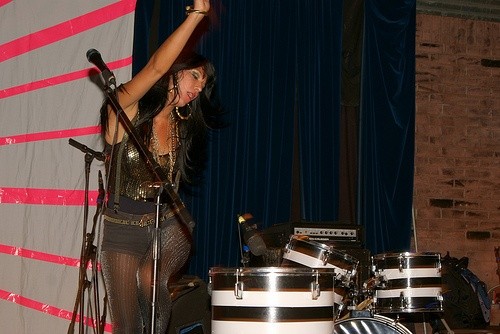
[
  {"xmin": 98, "ymin": 169, "xmax": 106, "ymax": 214},
  {"xmin": 237, "ymin": 214, "xmax": 267, "ymax": 256},
  {"xmin": 86, "ymin": 49, "xmax": 116, "ymax": 85}
]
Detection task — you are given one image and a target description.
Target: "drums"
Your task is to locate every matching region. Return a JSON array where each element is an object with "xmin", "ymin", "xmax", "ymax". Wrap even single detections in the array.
[
  {"xmin": 398, "ymin": 255, "xmax": 491, "ymax": 334},
  {"xmin": 279, "ymin": 234, "xmax": 359, "ymax": 315},
  {"xmin": 208, "ymin": 265, "xmax": 336, "ymax": 334},
  {"xmin": 333, "ymin": 314, "xmax": 413, "ymax": 334},
  {"xmin": 369, "ymin": 251, "xmax": 444, "ymax": 317}
]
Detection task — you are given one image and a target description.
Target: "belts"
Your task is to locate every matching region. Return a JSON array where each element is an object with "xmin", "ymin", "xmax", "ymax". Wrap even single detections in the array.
[{"xmin": 104, "ymin": 203, "xmax": 185, "ymax": 228}]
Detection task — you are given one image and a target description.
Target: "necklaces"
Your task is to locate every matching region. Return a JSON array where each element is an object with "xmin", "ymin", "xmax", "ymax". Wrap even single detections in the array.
[{"xmin": 150, "ymin": 112, "xmax": 176, "ymax": 183}]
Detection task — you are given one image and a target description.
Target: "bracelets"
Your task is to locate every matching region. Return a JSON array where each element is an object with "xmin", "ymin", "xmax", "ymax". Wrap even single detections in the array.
[{"xmin": 185, "ymin": 6, "xmax": 208, "ymax": 16}]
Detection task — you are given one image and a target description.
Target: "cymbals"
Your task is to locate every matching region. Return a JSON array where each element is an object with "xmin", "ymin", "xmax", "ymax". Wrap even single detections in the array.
[{"xmin": 246, "ymin": 220, "xmax": 361, "ymax": 257}]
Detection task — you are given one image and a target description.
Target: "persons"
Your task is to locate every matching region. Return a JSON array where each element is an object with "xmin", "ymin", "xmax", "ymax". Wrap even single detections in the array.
[{"xmin": 99, "ymin": 0, "xmax": 217, "ymax": 334}]
